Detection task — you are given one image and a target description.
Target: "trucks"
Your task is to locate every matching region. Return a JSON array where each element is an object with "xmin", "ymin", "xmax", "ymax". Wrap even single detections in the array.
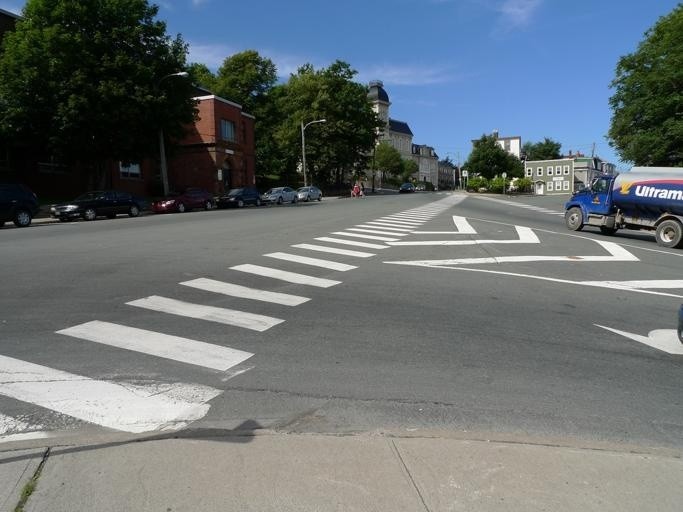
[{"xmin": 565, "ymin": 167, "xmax": 683, "ymax": 247}]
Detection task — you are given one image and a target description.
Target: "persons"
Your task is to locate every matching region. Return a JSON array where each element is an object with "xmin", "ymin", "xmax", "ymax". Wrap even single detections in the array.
[{"xmin": 348, "ymin": 174, "xmax": 369, "ymax": 199}]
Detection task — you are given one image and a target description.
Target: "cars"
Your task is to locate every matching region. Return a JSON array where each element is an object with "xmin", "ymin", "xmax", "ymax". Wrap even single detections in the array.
[
  {"xmin": 48, "ymin": 191, "xmax": 145, "ymax": 222},
  {"xmin": 151, "ymin": 188, "xmax": 214, "ymax": 212},
  {"xmin": 400, "ymin": 182, "xmax": 425, "ymax": 193},
  {"xmin": 216, "ymin": 186, "xmax": 322, "ymax": 208}
]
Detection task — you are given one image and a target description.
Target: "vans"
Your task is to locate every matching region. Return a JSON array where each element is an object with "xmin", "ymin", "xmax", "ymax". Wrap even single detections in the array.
[{"xmin": 0, "ymin": 183, "xmax": 41, "ymax": 227}]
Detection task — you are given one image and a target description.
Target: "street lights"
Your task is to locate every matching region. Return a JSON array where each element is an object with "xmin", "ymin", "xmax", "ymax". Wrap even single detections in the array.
[
  {"xmin": 157, "ymin": 72, "xmax": 189, "ymax": 196},
  {"xmin": 301, "ymin": 119, "xmax": 327, "ymax": 186},
  {"xmin": 372, "ymin": 134, "xmax": 384, "ymax": 192},
  {"xmin": 446, "ymin": 152, "xmax": 461, "ymax": 190}
]
